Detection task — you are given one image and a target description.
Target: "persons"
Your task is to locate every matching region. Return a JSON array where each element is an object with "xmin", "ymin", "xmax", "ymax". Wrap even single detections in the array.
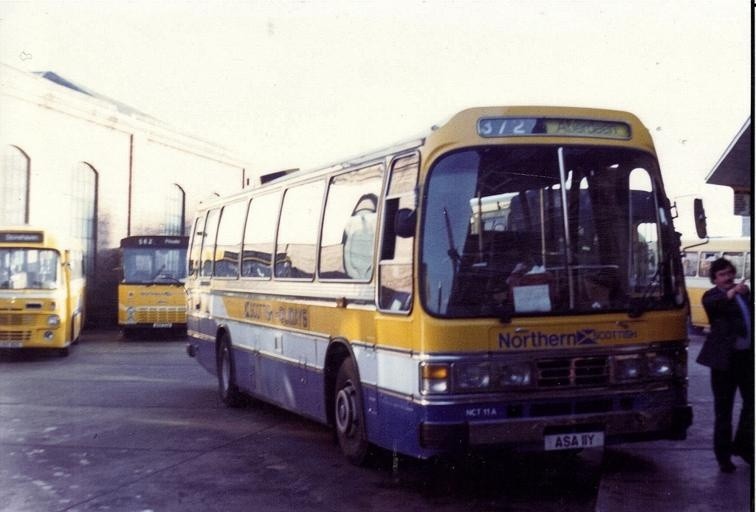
[{"xmin": 698, "ymin": 258, "xmax": 752, "ymax": 472}]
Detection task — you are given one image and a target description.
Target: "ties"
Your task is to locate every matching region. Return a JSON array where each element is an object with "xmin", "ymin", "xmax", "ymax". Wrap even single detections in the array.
[{"xmin": 734, "ymin": 297, "xmax": 747, "ymax": 339}]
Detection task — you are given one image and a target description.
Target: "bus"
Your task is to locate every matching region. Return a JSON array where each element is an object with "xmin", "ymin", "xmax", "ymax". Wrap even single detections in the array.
[
  {"xmin": 678, "ymin": 234, "xmax": 751, "ymax": 332},
  {"xmin": 0, "ymin": 225, "xmax": 88, "ymax": 357},
  {"xmin": 116, "ymin": 234, "xmax": 190, "ymax": 339}
]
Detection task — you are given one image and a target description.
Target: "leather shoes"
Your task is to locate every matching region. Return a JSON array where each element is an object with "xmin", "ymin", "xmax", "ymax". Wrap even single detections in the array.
[{"xmin": 718, "ymin": 455, "xmax": 736, "ymax": 473}]
